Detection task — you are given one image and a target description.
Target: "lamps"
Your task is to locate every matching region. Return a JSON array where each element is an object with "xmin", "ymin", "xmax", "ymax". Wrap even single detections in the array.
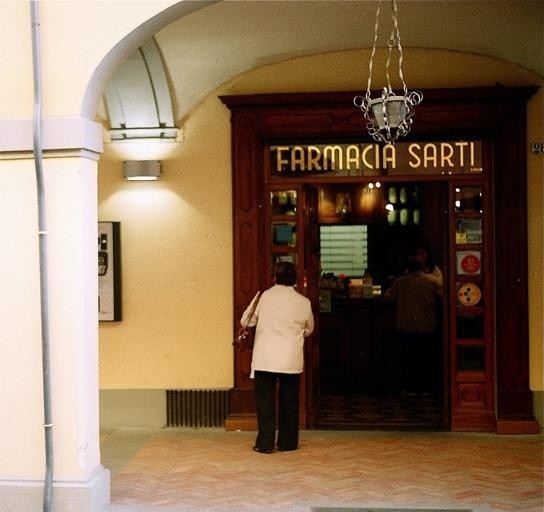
[
  {"xmin": 124, "ymin": 160, "xmax": 162, "ymax": 181},
  {"xmin": 353, "ymin": 0, "xmax": 423, "ymax": 145}
]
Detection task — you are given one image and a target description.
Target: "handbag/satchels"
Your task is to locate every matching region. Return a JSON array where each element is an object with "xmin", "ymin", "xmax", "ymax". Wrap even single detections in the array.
[{"xmin": 232, "ymin": 289, "xmax": 264, "ymax": 352}]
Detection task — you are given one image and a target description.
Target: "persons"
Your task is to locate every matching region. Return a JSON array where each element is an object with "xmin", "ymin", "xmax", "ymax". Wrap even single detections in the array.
[
  {"xmin": 382, "ymin": 257, "xmax": 444, "ymax": 397},
  {"xmin": 238, "ymin": 262, "xmax": 316, "ymax": 454},
  {"xmin": 405, "ymin": 247, "xmax": 443, "ymax": 283}
]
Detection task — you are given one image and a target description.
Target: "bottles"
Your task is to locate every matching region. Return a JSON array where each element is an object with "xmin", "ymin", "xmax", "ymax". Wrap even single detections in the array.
[
  {"xmin": 271, "ymin": 192, "xmax": 297, "ymax": 260},
  {"xmin": 386, "ymin": 185, "xmax": 427, "ymax": 225}
]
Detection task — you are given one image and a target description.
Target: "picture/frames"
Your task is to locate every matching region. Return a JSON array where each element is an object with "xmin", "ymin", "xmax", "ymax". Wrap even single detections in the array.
[{"xmin": 98, "ymin": 221, "xmax": 120, "ymax": 322}]
